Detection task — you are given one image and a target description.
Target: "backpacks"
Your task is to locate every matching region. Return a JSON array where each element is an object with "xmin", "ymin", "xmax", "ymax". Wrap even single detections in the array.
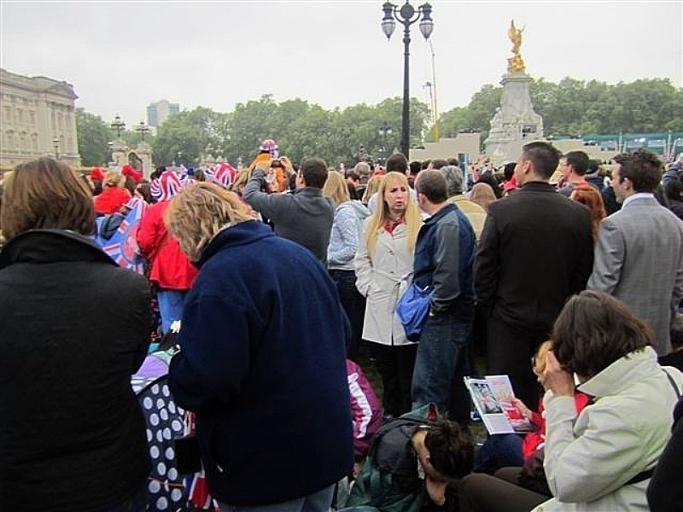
[{"xmin": 621, "ymin": 368, "xmax": 683, "ymax": 511}]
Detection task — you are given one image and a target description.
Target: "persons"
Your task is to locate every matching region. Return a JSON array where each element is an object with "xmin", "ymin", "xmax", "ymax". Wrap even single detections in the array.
[
  {"xmin": 345, "ymin": 180, "xmax": 359, "ymax": 201},
  {"xmin": 586, "ymin": 153, "xmax": 683, "ymax": 357},
  {"xmin": 0, "ymin": 157, "xmax": 153, "ymax": 511},
  {"xmin": 556, "ymin": 150, "xmax": 599, "ymax": 196},
  {"xmin": 458, "ymin": 289, "xmax": 683, "ymax": 511},
  {"xmin": 167, "ymin": 182, "xmax": 356, "ymax": 511},
  {"xmin": 586, "ymin": 160, "xmax": 618, "ymax": 216},
  {"xmin": 93, "ymin": 171, "xmax": 132, "ymax": 215},
  {"xmin": 474, "ymin": 142, "xmax": 595, "ymax": 407},
  {"xmin": 557, "ymin": 156, "xmax": 570, "ymax": 191},
  {"xmin": 467, "ymin": 164, "xmax": 501, "ymax": 199},
  {"xmin": 347, "ymin": 359, "xmax": 383, "ymax": 479},
  {"xmin": 342, "ymin": 420, "xmax": 475, "ymax": 512},
  {"xmin": 323, "ymin": 170, "xmax": 370, "ymax": 316},
  {"xmin": 241, "ymin": 157, "xmax": 335, "ymax": 265},
  {"xmin": 353, "ymin": 160, "xmax": 371, "ymax": 186},
  {"xmin": 137, "ymin": 172, "xmax": 203, "ymax": 334},
  {"xmin": 663, "ymin": 153, "xmax": 683, "ymax": 218},
  {"xmin": 249, "ymin": 140, "xmax": 284, "ymax": 194},
  {"xmin": 353, "ymin": 170, "xmax": 425, "ymax": 418},
  {"xmin": 438, "ymin": 166, "xmax": 487, "ymax": 240},
  {"xmin": 367, "ymin": 153, "xmax": 417, "ymax": 214},
  {"xmin": 373, "ymin": 166, "xmax": 385, "ymax": 176},
  {"xmin": 346, "ymin": 171, "xmax": 369, "ymax": 205},
  {"xmin": 410, "ymin": 169, "xmax": 478, "ymax": 416},
  {"xmin": 427, "ymin": 159, "xmax": 448, "ymax": 170},
  {"xmin": 472, "ymin": 340, "xmax": 590, "ymax": 470},
  {"xmin": 570, "ymin": 187, "xmax": 605, "ymax": 241}
]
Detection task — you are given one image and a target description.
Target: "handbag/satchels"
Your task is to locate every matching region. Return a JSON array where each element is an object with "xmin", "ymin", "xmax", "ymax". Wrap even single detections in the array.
[{"xmin": 394, "ymin": 211, "xmax": 478, "ymax": 342}]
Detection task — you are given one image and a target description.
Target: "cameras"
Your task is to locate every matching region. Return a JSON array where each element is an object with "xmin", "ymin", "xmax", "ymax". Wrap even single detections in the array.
[{"xmin": 271, "ymin": 159, "xmax": 282, "ymax": 168}]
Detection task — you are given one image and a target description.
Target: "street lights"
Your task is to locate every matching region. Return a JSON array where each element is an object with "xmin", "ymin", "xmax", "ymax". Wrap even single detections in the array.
[
  {"xmin": 133, "ymin": 122, "xmax": 152, "ymax": 142},
  {"xmin": 380, "ymin": 0, "xmax": 434, "ymax": 158},
  {"xmin": 109, "ymin": 113, "xmax": 126, "ymax": 138}
]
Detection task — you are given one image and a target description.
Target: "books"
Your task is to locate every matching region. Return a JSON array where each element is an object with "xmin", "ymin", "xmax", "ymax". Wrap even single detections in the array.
[{"xmin": 464, "ymin": 374, "xmax": 532, "ymax": 435}]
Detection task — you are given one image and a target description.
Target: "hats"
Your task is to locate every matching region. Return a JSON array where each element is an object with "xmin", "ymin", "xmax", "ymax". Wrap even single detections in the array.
[{"xmin": 260, "ymin": 139, "xmax": 279, "ymax": 151}]
